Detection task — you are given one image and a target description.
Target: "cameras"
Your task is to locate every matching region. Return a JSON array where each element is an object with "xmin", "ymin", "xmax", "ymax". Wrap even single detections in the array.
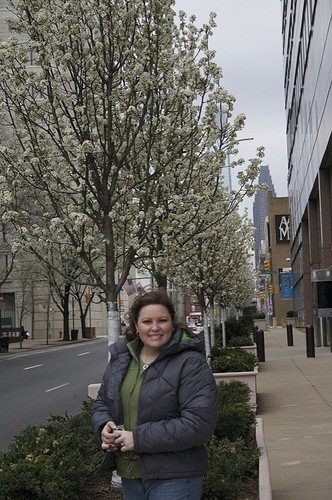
[{"xmin": 113, "ymin": 425, "xmax": 125, "ymax": 447}]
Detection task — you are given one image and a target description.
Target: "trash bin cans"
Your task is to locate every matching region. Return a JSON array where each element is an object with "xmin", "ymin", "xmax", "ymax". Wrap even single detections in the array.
[{"xmin": 317, "ymin": 317, "xmax": 327, "ymax": 347}]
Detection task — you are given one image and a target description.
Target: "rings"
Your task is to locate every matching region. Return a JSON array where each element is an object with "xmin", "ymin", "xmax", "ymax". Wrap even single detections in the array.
[{"xmin": 120, "ymin": 442, "xmax": 124, "ymax": 446}]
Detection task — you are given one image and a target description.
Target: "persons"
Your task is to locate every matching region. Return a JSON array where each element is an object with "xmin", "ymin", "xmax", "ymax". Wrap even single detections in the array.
[{"xmin": 92, "ymin": 292, "xmax": 217, "ymax": 500}]
